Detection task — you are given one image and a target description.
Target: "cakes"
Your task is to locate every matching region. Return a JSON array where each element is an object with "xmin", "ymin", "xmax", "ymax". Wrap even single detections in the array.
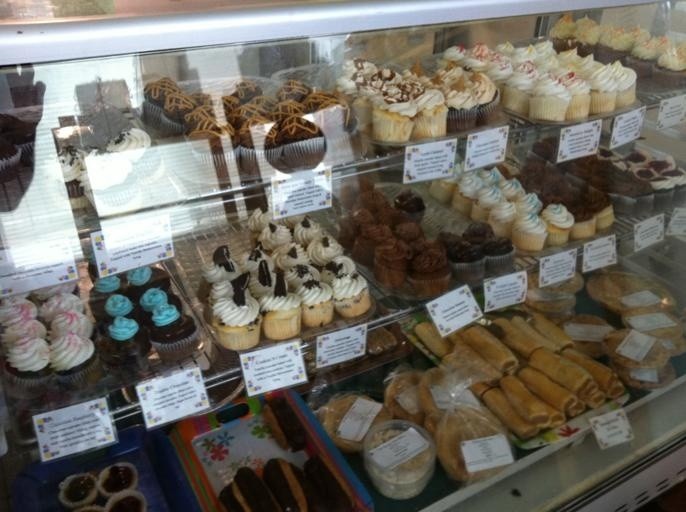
[
  {"xmin": 428, "ymin": 164, "xmax": 615, "ymax": 252},
  {"xmin": 334, "ymin": 59, "xmax": 500, "ymax": 143},
  {"xmin": 340, "ymin": 178, "xmax": 451, "ymax": 298},
  {"xmin": 58, "ymin": 462, "xmax": 147, "ymax": 512},
  {"xmin": 531, "ymin": 136, "xmax": 685, "ymax": 216},
  {"xmin": 0, "ymin": 282, "xmax": 100, "ymax": 401},
  {"xmin": 87, "ymin": 251, "xmax": 200, "ymax": 376},
  {"xmin": 40, "ymin": 109, "xmax": 166, "ymax": 217},
  {"xmin": 196, "ymin": 208, "xmax": 371, "ymax": 351},
  {"xmin": 436, "ymin": 222, "xmax": 517, "ymax": 281},
  {"xmin": 444, "ymin": 40, "xmax": 638, "ymax": 123},
  {"xmin": 0, "ymin": 111, "xmax": 38, "ymax": 182},
  {"xmin": 142, "ymin": 77, "xmax": 361, "ymax": 180},
  {"xmin": 550, "ymin": 14, "xmax": 686, "ymax": 88}
]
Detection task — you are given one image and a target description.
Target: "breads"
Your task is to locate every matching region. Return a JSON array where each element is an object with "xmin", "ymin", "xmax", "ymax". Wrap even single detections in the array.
[
  {"xmin": 522, "ymin": 271, "xmax": 685, "ymax": 389},
  {"xmin": 317, "ymin": 366, "xmax": 510, "ymax": 499},
  {"xmin": 217, "ymin": 458, "xmax": 356, "ymax": 511},
  {"xmin": 263, "ymin": 397, "xmax": 306, "ymax": 453},
  {"xmin": 414, "ymin": 314, "xmax": 626, "ymax": 440}
]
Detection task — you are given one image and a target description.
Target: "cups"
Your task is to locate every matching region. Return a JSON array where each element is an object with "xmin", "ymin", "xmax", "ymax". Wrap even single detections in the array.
[{"xmin": 0, "ymin": 13, "xmax": 686, "ymax": 401}]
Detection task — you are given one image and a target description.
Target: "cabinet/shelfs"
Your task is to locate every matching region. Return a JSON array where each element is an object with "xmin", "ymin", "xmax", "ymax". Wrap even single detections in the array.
[{"xmin": 0, "ymin": 0, "xmax": 686, "ymax": 512}]
